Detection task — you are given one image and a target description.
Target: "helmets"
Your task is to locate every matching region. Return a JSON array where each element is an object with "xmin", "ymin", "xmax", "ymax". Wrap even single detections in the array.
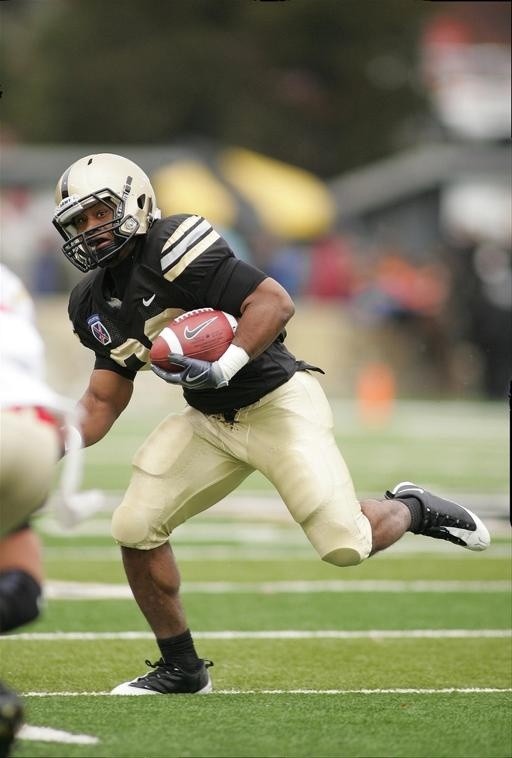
[{"xmin": 52, "ymin": 153, "xmax": 161, "ymax": 273}]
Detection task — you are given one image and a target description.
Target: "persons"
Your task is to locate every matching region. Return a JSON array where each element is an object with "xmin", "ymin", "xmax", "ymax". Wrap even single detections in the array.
[
  {"xmin": 0, "ymin": 264, "xmax": 106, "ymax": 758},
  {"xmin": 52, "ymin": 152, "xmax": 492, "ymax": 697}
]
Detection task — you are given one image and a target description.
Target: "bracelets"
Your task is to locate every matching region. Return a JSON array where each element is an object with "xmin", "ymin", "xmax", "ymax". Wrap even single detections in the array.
[{"xmin": 217, "ymin": 343, "xmax": 250, "ymax": 382}]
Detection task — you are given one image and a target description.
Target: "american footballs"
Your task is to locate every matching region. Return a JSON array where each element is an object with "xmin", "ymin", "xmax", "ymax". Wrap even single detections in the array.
[{"xmin": 149, "ymin": 307, "xmax": 239, "ymax": 373}]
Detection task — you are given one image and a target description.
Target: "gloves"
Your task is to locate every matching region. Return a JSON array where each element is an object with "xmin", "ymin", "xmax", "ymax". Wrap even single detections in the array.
[{"xmin": 149, "ymin": 353, "xmax": 229, "ymax": 390}]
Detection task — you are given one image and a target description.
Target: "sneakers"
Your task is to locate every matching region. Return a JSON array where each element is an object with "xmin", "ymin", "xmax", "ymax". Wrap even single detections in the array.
[
  {"xmin": 384, "ymin": 481, "xmax": 490, "ymax": 551},
  {"xmin": 110, "ymin": 657, "xmax": 213, "ymax": 696}
]
